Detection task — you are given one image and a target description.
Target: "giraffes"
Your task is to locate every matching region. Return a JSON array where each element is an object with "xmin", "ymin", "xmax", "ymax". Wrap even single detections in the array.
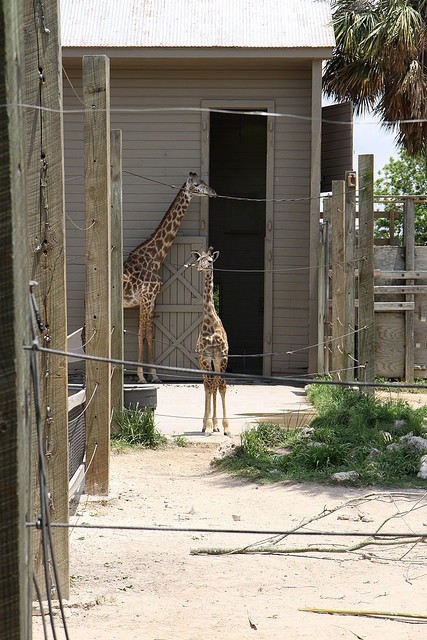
[
  {"xmin": 81, "ymin": 171, "xmax": 219, "ymax": 384},
  {"xmin": 189, "ymin": 244, "xmax": 230, "ymax": 437}
]
